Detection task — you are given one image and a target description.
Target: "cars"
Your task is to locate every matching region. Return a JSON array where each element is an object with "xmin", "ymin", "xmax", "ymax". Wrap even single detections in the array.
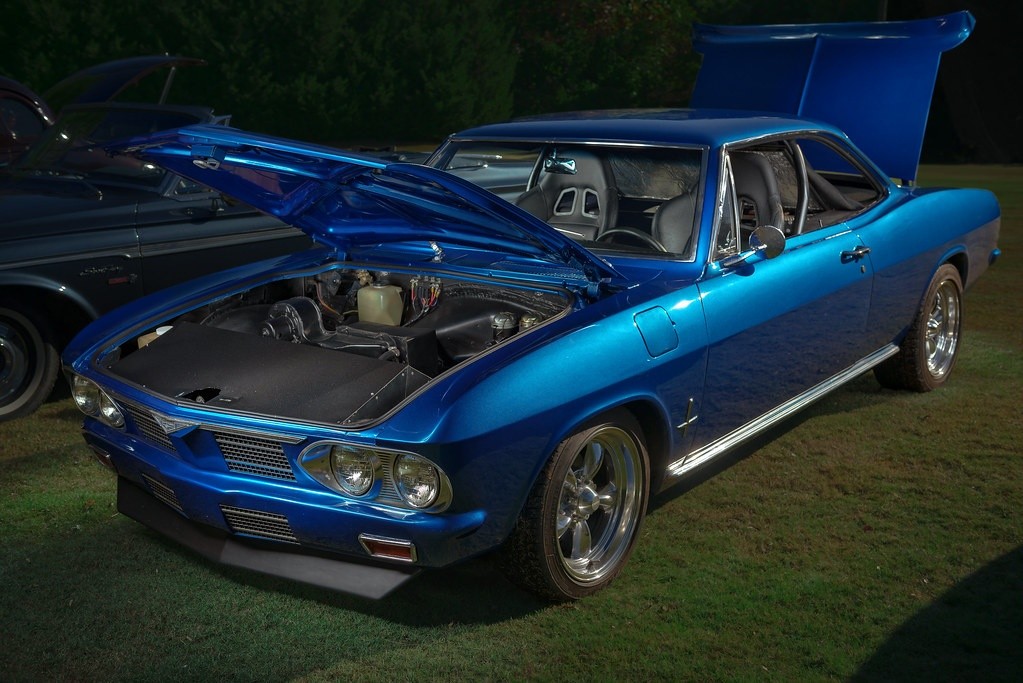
[
  {"xmin": 60, "ymin": 8, "xmax": 1003, "ymax": 603},
  {"xmin": 0, "ymin": 61, "xmax": 558, "ymax": 434}
]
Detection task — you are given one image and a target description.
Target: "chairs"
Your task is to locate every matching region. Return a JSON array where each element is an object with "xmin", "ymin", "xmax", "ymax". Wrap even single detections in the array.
[
  {"xmin": 512, "ymin": 151, "xmax": 620, "ymax": 241},
  {"xmin": 651, "ymin": 150, "xmax": 785, "ymax": 254}
]
